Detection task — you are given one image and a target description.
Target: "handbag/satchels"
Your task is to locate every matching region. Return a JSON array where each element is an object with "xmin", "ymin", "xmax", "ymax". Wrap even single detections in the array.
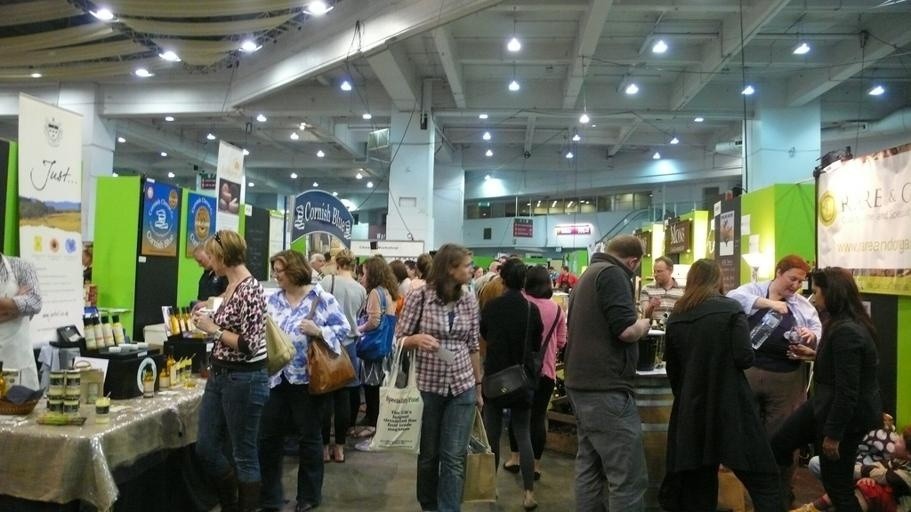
[
  {"xmin": 366, "ymin": 336, "xmax": 425, "ymax": 455},
  {"xmin": 265, "ymin": 314, "xmax": 296, "ymax": 375},
  {"xmin": 528, "ymin": 306, "xmax": 561, "ymax": 379},
  {"xmin": 309, "ymin": 296, "xmax": 355, "ymax": 394},
  {"xmin": 464, "ymin": 406, "xmax": 495, "ymax": 501},
  {"xmin": 482, "ymin": 302, "xmax": 531, "ymax": 407},
  {"xmin": 331, "ymin": 275, "xmax": 334, "ymax": 294},
  {"xmin": 396, "ymin": 292, "xmax": 425, "ymax": 387},
  {"xmin": 357, "ymin": 288, "xmax": 397, "ymax": 359},
  {"xmin": 489, "ymin": 275, "xmax": 495, "ymax": 281}
]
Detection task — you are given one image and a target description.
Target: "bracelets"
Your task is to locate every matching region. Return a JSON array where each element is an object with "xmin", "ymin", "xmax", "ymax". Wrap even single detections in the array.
[{"xmin": 474, "ymin": 381, "xmax": 482, "ymax": 385}]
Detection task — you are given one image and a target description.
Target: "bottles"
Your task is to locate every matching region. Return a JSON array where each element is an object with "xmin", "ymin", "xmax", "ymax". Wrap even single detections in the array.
[
  {"xmin": 168, "ymin": 308, "xmax": 180, "ymax": 335},
  {"xmin": 0, "ymin": 360, "xmax": 5, "ymax": 398},
  {"xmin": 111, "ymin": 315, "xmax": 125, "ymax": 346},
  {"xmin": 90, "ymin": 316, "xmax": 105, "ymax": 348},
  {"xmin": 173, "ymin": 307, "xmax": 187, "ymax": 333},
  {"xmin": 48, "ymin": 396, "xmax": 63, "ymax": 417},
  {"xmin": 64, "ymin": 369, "xmax": 80, "ymax": 395},
  {"xmin": 46, "ymin": 394, "xmax": 48, "ymax": 415},
  {"xmin": 48, "ymin": 369, "xmax": 64, "ymax": 395},
  {"xmin": 77, "ymin": 395, "xmax": 80, "ymax": 417},
  {"xmin": 63, "ymin": 395, "xmax": 78, "ymax": 418},
  {"xmin": 142, "ymin": 364, "xmax": 154, "ymax": 398},
  {"xmin": 165, "ymin": 346, "xmax": 175, "ymax": 387},
  {"xmin": 185, "ymin": 307, "xmax": 197, "ymax": 331},
  {"xmin": 180, "ymin": 307, "xmax": 192, "ymax": 332},
  {"xmin": 429, "ymin": 344, "xmax": 454, "ymax": 364},
  {"xmin": 100, "ymin": 316, "xmax": 114, "ymax": 346},
  {"xmin": 748, "ymin": 308, "xmax": 783, "ymax": 349},
  {"xmin": 158, "ymin": 360, "xmax": 170, "ymax": 392},
  {"xmin": 82, "ymin": 318, "xmax": 96, "ymax": 349}
]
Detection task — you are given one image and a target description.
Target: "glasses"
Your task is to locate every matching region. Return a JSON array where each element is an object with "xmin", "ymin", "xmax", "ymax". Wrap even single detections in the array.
[{"xmin": 276, "ymin": 268, "xmax": 285, "ymax": 273}]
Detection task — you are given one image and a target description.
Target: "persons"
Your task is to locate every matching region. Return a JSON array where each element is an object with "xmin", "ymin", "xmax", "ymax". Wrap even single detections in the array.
[
  {"xmin": 82, "ymin": 244, "xmax": 94, "ymax": 287},
  {"xmin": 411, "ymin": 254, "xmax": 432, "ymax": 289},
  {"xmin": 666, "ymin": 258, "xmax": 785, "ymax": 511},
  {"xmin": 787, "ymin": 294, "xmax": 819, "ymax": 397},
  {"xmin": 261, "ymin": 250, "xmax": 351, "ymax": 512},
  {"xmin": 338, "ymin": 353, "xmax": 362, "ymax": 440},
  {"xmin": 318, "ymin": 249, "xmax": 361, "ymax": 463},
  {"xmin": 641, "ymin": 258, "xmax": 684, "ymax": 361},
  {"xmin": 404, "ymin": 261, "xmax": 418, "ymax": 280},
  {"xmin": 479, "ymin": 260, "xmax": 544, "ymax": 509},
  {"xmin": 725, "ymin": 255, "xmax": 822, "ymax": 511},
  {"xmin": 1, "ymin": 255, "xmax": 41, "ymax": 392},
  {"xmin": 472, "ymin": 252, "xmax": 507, "ymax": 300},
  {"xmin": 356, "ymin": 257, "xmax": 397, "ymax": 437},
  {"xmin": 809, "ymin": 413, "xmax": 900, "ymax": 481},
  {"xmin": 309, "ymin": 248, "xmax": 335, "ymax": 278},
  {"xmin": 395, "ymin": 243, "xmax": 483, "ymax": 512},
  {"xmin": 190, "ymin": 243, "xmax": 228, "ymax": 306},
  {"xmin": 191, "ymin": 232, "xmax": 269, "ymax": 512},
  {"xmin": 504, "ymin": 266, "xmax": 566, "ymax": 480},
  {"xmin": 565, "ymin": 234, "xmax": 650, "ymax": 511},
  {"xmin": 772, "ymin": 267, "xmax": 885, "ymax": 512},
  {"xmin": 356, "ymin": 265, "xmax": 366, "ymax": 287},
  {"xmin": 793, "ymin": 428, "xmax": 909, "ymax": 511},
  {"xmin": 389, "ymin": 260, "xmax": 413, "ymax": 373},
  {"xmin": 528, "ymin": 257, "xmax": 576, "ymax": 292}
]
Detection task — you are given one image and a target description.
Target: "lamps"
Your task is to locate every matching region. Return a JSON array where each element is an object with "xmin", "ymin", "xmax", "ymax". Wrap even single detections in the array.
[
  {"xmin": 670, "ymin": 112, "xmax": 680, "ymax": 145},
  {"xmin": 739, "ymin": 64, "xmax": 755, "ymax": 99},
  {"xmin": 302, "ymin": 0, "xmax": 332, "ymax": 20},
  {"xmin": 564, "ymin": 135, "xmax": 577, "ymax": 161},
  {"xmin": 252, "ymin": 112, "xmax": 267, "ymax": 124},
  {"xmin": 205, "ymin": 131, "xmax": 217, "ymax": 142},
  {"xmin": 339, "ymin": 17, "xmax": 358, "ymax": 90},
  {"xmin": 238, "ymin": 35, "xmax": 262, "ymax": 55},
  {"xmin": 792, "ymin": 10, "xmax": 812, "ymax": 56},
  {"xmin": 87, "ymin": 6, "xmax": 114, "ymax": 24},
  {"xmin": 650, "ymin": 13, "xmax": 668, "ymax": 58},
  {"xmin": 572, "ymin": 123, "xmax": 582, "ymax": 143},
  {"xmin": 693, "ymin": 97, "xmax": 704, "ymax": 124},
  {"xmin": 134, "ymin": 65, "xmax": 151, "ymax": 79},
  {"xmin": 287, "ymin": 130, "xmax": 299, "ymax": 142},
  {"xmin": 579, "ymin": 82, "xmax": 591, "ymax": 128},
  {"xmin": 158, "ymin": 48, "xmax": 179, "ymax": 63},
  {"xmin": 578, "ymin": 51, "xmax": 590, "ymax": 124},
  {"xmin": 865, "ymin": 59, "xmax": 885, "ymax": 99},
  {"xmin": 507, "ymin": 55, "xmax": 521, "ymax": 91},
  {"xmin": 362, "ymin": 95, "xmax": 375, "ymax": 123},
  {"xmin": 506, "ymin": 5, "xmax": 522, "ymax": 54}
]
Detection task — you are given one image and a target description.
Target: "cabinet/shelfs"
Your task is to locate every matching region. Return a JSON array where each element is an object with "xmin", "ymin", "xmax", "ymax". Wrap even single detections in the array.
[{"xmin": 0, "ymin": 347, "xmax": 216, "ymax": 512}]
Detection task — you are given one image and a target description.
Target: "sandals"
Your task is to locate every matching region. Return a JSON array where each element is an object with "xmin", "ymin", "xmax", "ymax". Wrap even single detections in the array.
[
  {"xmin": 353, "ymin": 427, "xmax": 375, "ymax": 437},
  {"xmin": 347, "ymin": 425, "xmax": 366, "ymax": 435}
]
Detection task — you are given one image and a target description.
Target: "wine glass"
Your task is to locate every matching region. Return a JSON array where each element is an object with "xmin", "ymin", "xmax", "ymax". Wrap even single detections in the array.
[{"xmin": 790, "ymin": 327, "xmax": 802, "ymax": 360}]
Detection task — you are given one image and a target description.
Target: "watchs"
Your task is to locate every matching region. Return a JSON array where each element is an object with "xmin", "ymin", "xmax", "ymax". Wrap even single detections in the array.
[{"xmin": 212, "ymin": 325, "xmax": 226, "ymax": 341}]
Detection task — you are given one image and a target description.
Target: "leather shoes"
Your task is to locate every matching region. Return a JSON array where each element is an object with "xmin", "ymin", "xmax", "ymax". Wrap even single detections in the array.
[
  {"xmin": 503, "ymin": 462, "xmax": 520, "ymax": 472},
  {"xmin": 255, "ymin": 507, "xmax": 274, "ymax": 511},
  {"xmin": 496, "ymin": 495, "xmax": 498, "ymax": 499},
  {"xmin": 536, "ymin": 472, "xmax": 540, "ymax": 478},
  {"xmin": 337, "ymin": 454, "xmax": 345, "ymax": 462},
  {"xmin": 296, "ymin": 502, "xmax": 312, "ymax": 512},
  {"xmin": 324, "ymin": 460, "xmax": 330, "ymax": 462},
  {"xmin": 525, "ymin": 504, "xmax": 537, "ymax": 511}
]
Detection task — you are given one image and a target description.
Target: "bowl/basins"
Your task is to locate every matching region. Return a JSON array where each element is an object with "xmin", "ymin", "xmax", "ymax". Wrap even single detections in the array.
[{"xmin": 784, "ymin": 331, "xmax": 807, "ymax": 344}]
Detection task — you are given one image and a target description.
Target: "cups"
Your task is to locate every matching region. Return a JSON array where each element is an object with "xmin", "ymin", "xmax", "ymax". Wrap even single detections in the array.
[
  {"xmin": 143, "ymin": 380, "xmax": 155, "ymax": 398},
  {"xmin": 169, "ymin": 357, "xmax": 195, "ymax": 388},
  {"xmin": 95, "ymin": 397, "xmax": 112, "ymax": 425},
  {"xmin": 159, "ymin": 369, "xmax": 171, "ymax": 393}
]
